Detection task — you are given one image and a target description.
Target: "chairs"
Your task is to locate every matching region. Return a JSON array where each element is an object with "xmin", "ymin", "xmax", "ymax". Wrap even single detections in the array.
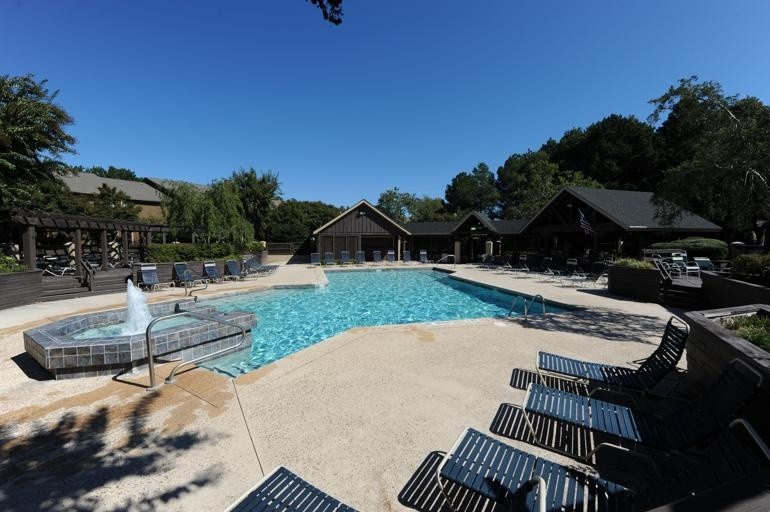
[
  {"xmin": 36, "ymin": 254, "xmax": 118, "ymax": 277},
  {"xmin": 310, "ymin": 249, "xmax": 429, "ymax": 265},
  {"xmin": 230, "ymin": 466, "xmax": 361, "ymax": 512},
  {"xmin": 524, "ymin": 358, "xmax": 762, "ymax": 450},
  {"xmin": 481, "ymin": 253, "xmax": 585, "ymax": 277},
  {"xmin": 436, "ymin": 418, "xmax": 769, "ymax": 512},
  {"xmin": 137, "ymin": 261, "xmax": 223, "ymax": 293},
  {"xmin": 536, "ymin": 317, "xmax": 690, "ymax": 409},
  {"xmin": 652, "ymin": 254, "xmax": 714, "ymax": 279},
  {"xmin": 225, "ymin": 260, "xmax": 247, "ymax": 281},
  {"xmin": 244, "ymin": 258, "xmax": 279, "ymax": 273}
]
